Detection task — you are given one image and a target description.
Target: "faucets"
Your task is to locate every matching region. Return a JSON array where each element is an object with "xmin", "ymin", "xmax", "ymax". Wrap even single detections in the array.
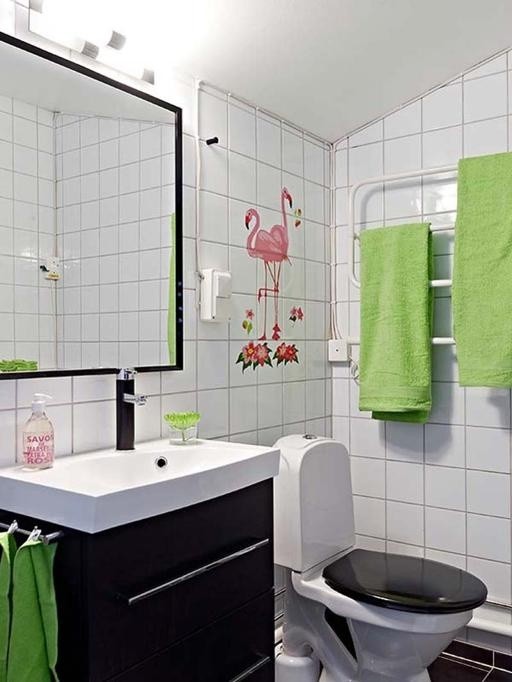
[{"xmin": 116, "ymin": 366, "xmax": 148, "ymax": 451}]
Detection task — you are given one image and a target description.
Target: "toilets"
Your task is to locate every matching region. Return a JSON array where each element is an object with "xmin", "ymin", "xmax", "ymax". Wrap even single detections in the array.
[{"xmin": 272, "ymin": 432, "xmax": 488, "ymax": 681}]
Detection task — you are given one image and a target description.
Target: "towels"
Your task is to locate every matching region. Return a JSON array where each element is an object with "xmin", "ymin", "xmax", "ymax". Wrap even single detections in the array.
[
  {"xmin": 8, "ymin": 538, "xmax": 59, "ymax": 682},
  {"xmin": 0, "ymin": 531, "xmax": 17, "ymax": 682},
  {"xmin": 359, "ymin": 223, "xmax": 432, "ymax": 424},
  {"xmin": 1, "ymin": 359, "xmax": 37, "ymax": 371},
  {"xmin": 167, "ymin": 213, "xmax": 176, "ymax": 366},
  {"xmin": 451, "ymin": 153, "xmax": 512, "ymax": 391}
]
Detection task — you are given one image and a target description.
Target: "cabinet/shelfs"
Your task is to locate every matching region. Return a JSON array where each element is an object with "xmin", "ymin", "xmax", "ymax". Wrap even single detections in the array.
[{"xmin": 348, "ymin": 165, "xmax": 458, "ymax": 345}]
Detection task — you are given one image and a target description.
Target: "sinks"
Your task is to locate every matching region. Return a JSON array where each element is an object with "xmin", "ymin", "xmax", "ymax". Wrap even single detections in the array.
[{"xmin": 1, "ymin": 437, "xmax": 281, "ymax": 534}]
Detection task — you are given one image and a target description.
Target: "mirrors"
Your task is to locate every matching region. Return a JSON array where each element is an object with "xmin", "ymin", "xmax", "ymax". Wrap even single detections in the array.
[{"xmin": 1, "ymin": 33, "xmax": 184, "ymax": 381}]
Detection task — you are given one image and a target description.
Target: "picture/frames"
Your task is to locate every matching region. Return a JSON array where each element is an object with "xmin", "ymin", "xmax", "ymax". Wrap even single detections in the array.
[{"xmin": 238, "ymin": 187, "xmax": 305, "ymax": 370}]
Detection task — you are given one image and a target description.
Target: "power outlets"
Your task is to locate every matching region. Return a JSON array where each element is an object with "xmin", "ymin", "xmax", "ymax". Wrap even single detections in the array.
[{"xmin": 327, "ymin": 340, "xmax": 347, "ymax": 361}]
